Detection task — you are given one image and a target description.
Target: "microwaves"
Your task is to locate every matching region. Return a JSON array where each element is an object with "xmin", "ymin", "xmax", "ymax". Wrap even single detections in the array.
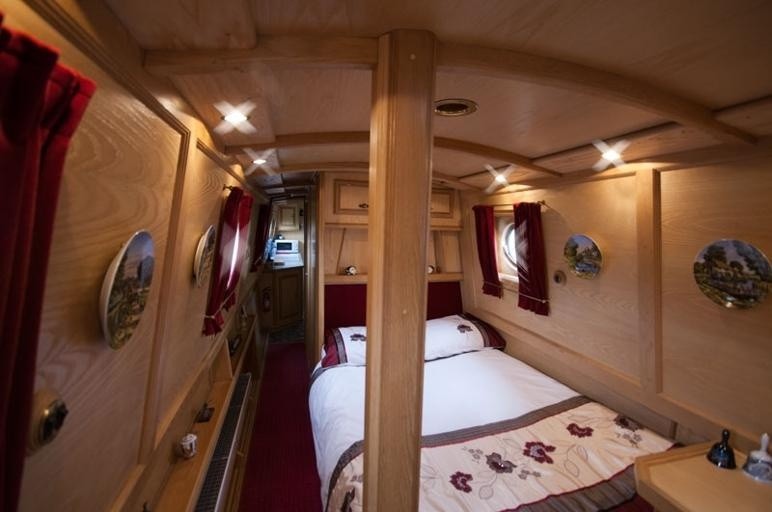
[{"xmin": 275, "ymin": 239, "xmax": 299, "ymax": 254}]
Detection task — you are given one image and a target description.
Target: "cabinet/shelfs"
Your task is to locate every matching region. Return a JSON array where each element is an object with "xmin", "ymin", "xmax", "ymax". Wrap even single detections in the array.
[
  {"xmin": 320, "ymin": 170, "xmax": 463, "ymax": 229},
  {"xmin": 103, "ymin": 273, "xmax": 257, "ymax": 512},
  {"xmin": 255, "ymin": 268, "xmax": 304, "ymax": 333}
]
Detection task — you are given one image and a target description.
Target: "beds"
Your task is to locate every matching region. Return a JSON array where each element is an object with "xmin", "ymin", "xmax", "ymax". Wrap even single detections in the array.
[{"xmin": 307, "ymin": 317, "xmax": 685, "ymax": 509}]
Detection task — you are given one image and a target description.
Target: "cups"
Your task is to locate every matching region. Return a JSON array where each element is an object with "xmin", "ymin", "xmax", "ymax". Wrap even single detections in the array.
[{"xmin": 179, "ymin": 434, "xmax": 198, "ymax": 458}]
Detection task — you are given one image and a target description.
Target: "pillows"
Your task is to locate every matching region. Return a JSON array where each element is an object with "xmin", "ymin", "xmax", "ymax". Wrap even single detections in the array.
[{"xmin": 324, "ymin": 313, "xmax": 503, "ymax": 366}]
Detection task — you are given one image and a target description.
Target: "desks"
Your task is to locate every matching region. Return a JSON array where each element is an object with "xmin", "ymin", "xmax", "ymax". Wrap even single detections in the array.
[{"xmin": 636, "ymin": 439, "xmax": 770, "ymax": 511}]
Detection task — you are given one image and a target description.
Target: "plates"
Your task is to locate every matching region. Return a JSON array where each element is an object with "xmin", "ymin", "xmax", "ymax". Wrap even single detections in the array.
[
  {"xmin": 564, "ymin": 234, "xmax": 602, "ymax": 281},
  {"xmin": 98, "ymin": 229, "xmax": 155, "ymax": 352},
  {"xmin": 193, "ymin": 224, "xmax": 218, "ymax": 288},
  {"xmin": 693, "ymin": 240, "xmax": 772, "ymax": 310}
]
